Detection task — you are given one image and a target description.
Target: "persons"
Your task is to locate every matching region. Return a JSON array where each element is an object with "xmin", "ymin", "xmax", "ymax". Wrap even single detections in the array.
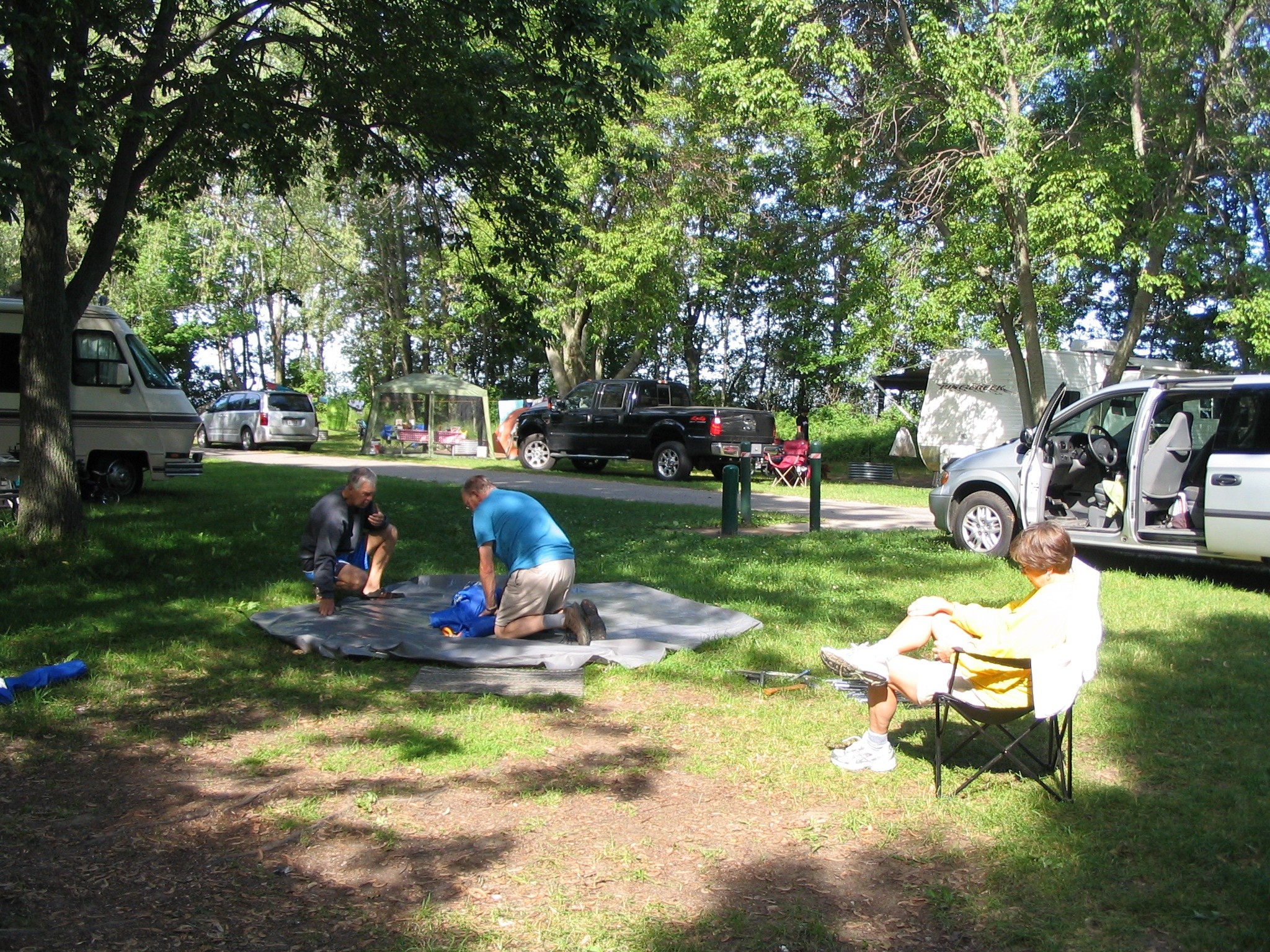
[
  {"xmin": 819, "ymin": 521, "xmax": 1103, "ymax": 769},
  {"xmin": 301, "ymin": 467, "xmax": 405, "ymax": 616},
  {"xmin": 796, "ymin": 407, "xmax": 809, "ymax": 441},
  {"xmin": 462, "ymin": 475, "xmax": 606, "ymax": 646}
]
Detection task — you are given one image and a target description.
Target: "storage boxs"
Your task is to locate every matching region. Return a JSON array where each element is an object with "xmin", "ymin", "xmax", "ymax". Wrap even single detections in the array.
[
  {"xmin": 397, "ymin": 429, "xmax": 428, "ymax": 441},
  {"xmin": 434, "ymin": 431, "xmax": 463, "ymax": 442}
]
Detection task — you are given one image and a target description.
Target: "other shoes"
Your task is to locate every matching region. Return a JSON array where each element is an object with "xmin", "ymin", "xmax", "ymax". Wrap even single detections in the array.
[
  {"xmin": 361, "ymin": 586, "xmax": 404, "ymax": 601},
  {"xmin": 312, "ymin": 581, "xmax": 324, "ymax": 603}
]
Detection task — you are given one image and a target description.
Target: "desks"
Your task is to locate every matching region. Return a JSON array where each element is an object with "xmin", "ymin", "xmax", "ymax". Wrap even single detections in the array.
[{"xmin": 0, "ymin": 456, "xmax": 20, "ymax": 521}]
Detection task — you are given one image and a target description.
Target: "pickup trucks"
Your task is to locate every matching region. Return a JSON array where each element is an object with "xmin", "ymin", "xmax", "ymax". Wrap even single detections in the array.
[{"xmin": 512, "ymin": 376, "xmax": 786, "ymax": 482}]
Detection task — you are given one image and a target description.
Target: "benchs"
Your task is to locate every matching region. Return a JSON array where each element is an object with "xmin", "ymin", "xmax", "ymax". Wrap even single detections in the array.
[
  {"xmin": 0, "ymin": 488, "xmax": 20, "ymax": 498},
  {"xmin": 396, "ymin": 441, "xmax": 461, "ymax": 457}
]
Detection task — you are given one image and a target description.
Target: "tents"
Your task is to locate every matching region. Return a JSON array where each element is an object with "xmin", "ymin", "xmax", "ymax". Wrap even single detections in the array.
[{"xmin": 365, "ymin": 372, "xmax": 495, "ymax": 459}]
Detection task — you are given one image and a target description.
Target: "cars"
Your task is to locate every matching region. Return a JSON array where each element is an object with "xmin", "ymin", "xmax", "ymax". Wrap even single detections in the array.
[{"xmin": 196, "ymin": 388, "xmax": 319, "ymax": 450}]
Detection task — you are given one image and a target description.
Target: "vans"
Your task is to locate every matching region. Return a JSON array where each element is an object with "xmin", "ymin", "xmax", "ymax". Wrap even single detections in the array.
[
  {"xmin": 926, "ymin": 365, "xmax": 1270, "ymax": 570},
  {"xmin": 0, "ymin": 293, "xmax": 206, "ymax": 501},
  {"xmin": 917, "ymin": 345, "xmax": 1225, "ymax": 480}
]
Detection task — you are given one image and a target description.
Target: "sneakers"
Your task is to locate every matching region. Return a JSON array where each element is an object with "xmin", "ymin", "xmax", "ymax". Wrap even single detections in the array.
[
  {"xmin": 831, "ymin": 735, "xmax": 897, "ymax": 772},
  {"xmin": 578, "ymin": 599, "xmax": 606, "ymax": 640},
  {"xmin": 559, "ymin": 601, "xmax": 591, "ymax": 645},
  {"xmin": 820, "ymin": 642, "xmax": 889, "ymax": 686}
]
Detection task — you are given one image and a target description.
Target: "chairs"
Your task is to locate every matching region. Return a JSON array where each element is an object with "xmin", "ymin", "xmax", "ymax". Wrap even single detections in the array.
[
  {"xmin": 766, "ymin": 440, "xmax": 809, "ymax": 488},
  {"xmin": 1094, "ymin": 411, "xmax": 1192, "ymax": 511},
  {"xmin": 932, "ymin": 647, "xmax": 1074, "ymax": 804}
]
